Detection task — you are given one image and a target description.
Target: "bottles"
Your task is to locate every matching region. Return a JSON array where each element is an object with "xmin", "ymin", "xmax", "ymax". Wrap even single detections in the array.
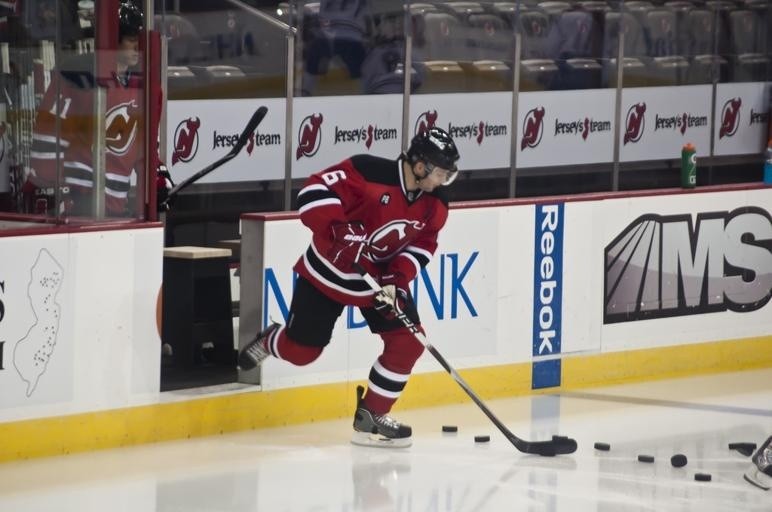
[
  {"xmin": 680, "ymin": 141, "xmax": 696, "ymax": 189},
  {"xmin": 763, "ymin": 136, "xmax": 772, "ymax": 186}
]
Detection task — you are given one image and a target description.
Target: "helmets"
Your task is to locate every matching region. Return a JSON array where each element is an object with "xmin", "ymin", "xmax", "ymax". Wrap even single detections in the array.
[
  {"xmin": 118, "ymin": 1, "xmax": 145, "ymax": 44},
  {"xmin": 407, "ymin": 126, "xmax": 461, "ymax": 187}
]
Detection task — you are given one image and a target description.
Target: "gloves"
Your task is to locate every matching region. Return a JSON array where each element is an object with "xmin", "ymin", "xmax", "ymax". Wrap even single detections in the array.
[
  {"xmin": 331, "ymin": 221, "xmax": 369, "ymax": 247},
  {"xmin": 157, "ymin": 164, "xmax": 178, "ymax": 212},
  {"xmin": 34, "ymin": 185, "xmax": 75, "ymax": 216},
  {"xmin": 371, "ymin": 270, "xmax": 410, "ymax": 321}
]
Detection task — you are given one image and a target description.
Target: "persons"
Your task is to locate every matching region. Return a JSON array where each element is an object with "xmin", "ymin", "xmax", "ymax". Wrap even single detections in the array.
[
  {"xmin": 362, "ymin": 12, "xmax": 431, "ymax": 96},
  {"xmin": 20, "ymin": 1, "xmax": 177, "ymax": 219},
  {"xmin": 239, "ymin": 125, "xmax": 461, "ymax": 441},
  {"xmin": 540, "ymin": 1, "xmax": 623, "ymax": 90},
  {"xmin": 301, "ymin": 1, "xmax": 378, "ymax": 87}
]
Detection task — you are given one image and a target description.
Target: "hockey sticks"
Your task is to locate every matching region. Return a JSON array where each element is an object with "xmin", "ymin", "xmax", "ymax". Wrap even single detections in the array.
[
  {"xmin": 164, "ymin": 106, "xmax": 268, "ymax": 202},
  {"xmin": 353, "ymin": 263, "xmax": 577, "ymax": 453}
]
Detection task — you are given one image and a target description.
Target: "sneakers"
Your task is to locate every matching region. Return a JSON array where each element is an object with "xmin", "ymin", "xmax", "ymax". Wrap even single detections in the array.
[
  {"xmin": 234, "ymin": 322, "xmax": 282, "ymax": 373},
  {"xmin": 349, "ymin": 385, "xmax": 413, "ymax": 448}
]
